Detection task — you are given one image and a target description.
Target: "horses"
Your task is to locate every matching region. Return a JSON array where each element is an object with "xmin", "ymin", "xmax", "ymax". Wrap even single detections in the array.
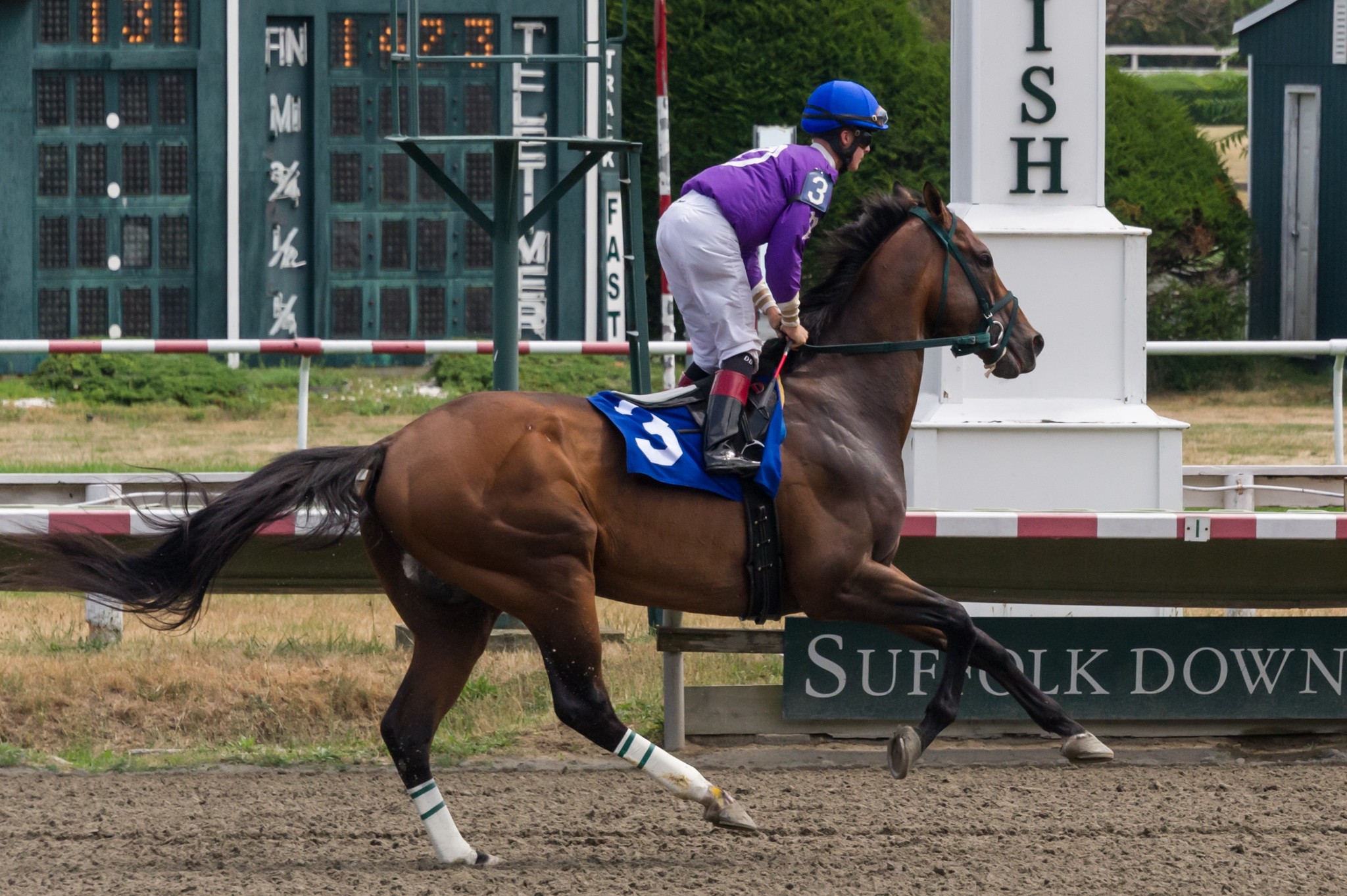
[{"xmin": 0, "ymin": 180, "xmax": 1120, "ymax": 867}]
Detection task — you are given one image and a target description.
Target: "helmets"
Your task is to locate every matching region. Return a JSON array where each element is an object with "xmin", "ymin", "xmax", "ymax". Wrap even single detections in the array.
[{"xmin": 801, "ymin": 80, "xmax": 889, "ymax": 133}]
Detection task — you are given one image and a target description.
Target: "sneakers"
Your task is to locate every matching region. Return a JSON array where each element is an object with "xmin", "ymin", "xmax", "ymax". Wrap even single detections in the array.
[{"xmin": 704, "ymin": 439, "xmax": 761, "ymax": 478}]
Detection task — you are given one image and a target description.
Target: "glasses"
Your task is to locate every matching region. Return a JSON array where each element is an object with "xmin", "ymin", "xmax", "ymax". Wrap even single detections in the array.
[{"xmin": 855, "ymin": 129, "xmax": 872, "ymax": 149}]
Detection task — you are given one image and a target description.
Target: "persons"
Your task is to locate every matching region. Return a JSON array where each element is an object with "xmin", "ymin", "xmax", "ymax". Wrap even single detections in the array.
[{"xmin": 655, "ymin": 81, "xmax": 888, "ymax": 467}]
